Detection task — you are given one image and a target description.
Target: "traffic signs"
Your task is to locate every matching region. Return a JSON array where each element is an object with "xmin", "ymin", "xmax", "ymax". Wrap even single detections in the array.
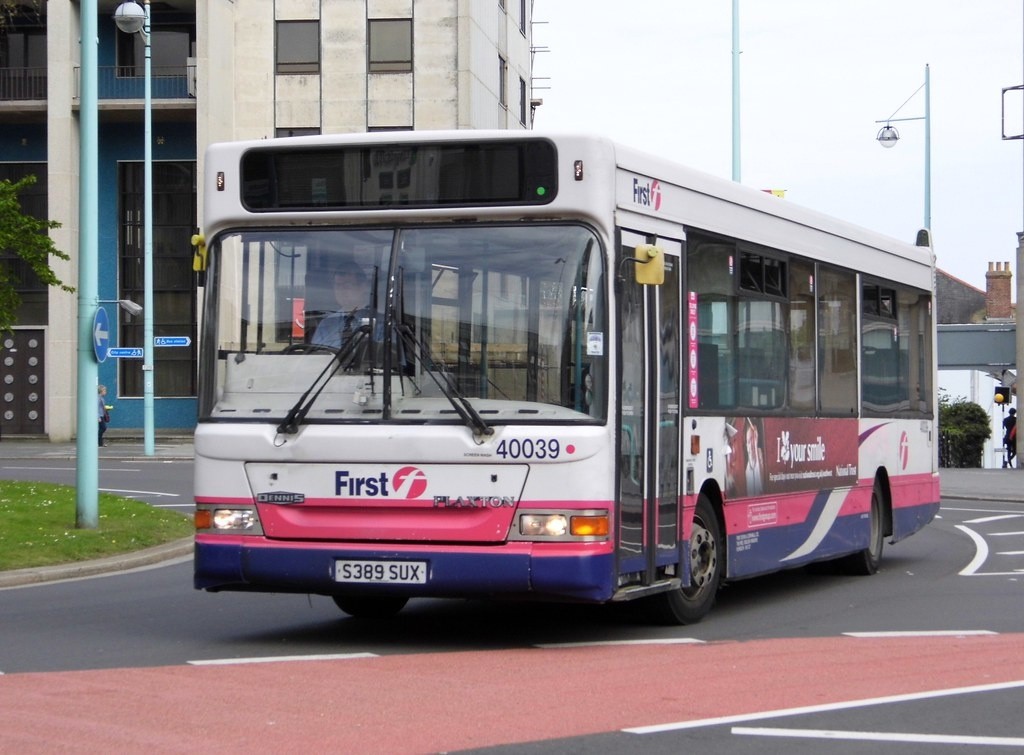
[
  {"xmin": 104, "ymin": 347, "xmax": 144, "ymax": 360},
  {"xmin": 152, "ymin": 336, "xmax": 192, "ymax": 347}
]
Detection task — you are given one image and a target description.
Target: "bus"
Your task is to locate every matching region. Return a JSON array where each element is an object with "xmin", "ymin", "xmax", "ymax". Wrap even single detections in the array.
[{"xmin": 190, "ymin": 130, "xmax": 943, "ymax": 625}]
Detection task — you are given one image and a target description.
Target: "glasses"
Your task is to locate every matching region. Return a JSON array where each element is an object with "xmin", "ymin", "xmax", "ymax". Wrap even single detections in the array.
[{"xmin": 334, "ymin": 281, "xmax": 359, "ymax": 289}]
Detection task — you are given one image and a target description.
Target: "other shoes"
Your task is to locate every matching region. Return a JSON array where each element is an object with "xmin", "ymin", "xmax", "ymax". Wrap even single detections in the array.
[{"xmin": 1002, "ymin": 460, "xmax": 1007, "ymax": 468}]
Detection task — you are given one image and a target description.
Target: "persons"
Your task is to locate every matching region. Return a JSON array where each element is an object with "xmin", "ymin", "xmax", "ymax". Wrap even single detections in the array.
[
  {"xmin": 98, "ymin": 385, "xmax": 113, "ymax": 446},
  {"xmin": 312, "ymin": 262, "xmax": 406, "ymax": 373},
  {"xmin": 1001, "ymin": 407, "xmax": 1018, "ymax": 468}
]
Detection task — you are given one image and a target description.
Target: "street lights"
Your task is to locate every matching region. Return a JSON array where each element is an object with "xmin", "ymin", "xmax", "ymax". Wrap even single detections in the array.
[
  {"xmin": 875, "ymin": 63, "xmax": 931, "ymax": 235},
  {"xmin": 114, "ymin": 0, "xmax": 155, "ymax": 457}
]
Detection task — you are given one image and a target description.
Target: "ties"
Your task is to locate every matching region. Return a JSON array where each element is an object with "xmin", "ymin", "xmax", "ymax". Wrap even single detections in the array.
[{"xmin": 341, "ymin": 315, "xmax": 354, "ymax": 348}]
